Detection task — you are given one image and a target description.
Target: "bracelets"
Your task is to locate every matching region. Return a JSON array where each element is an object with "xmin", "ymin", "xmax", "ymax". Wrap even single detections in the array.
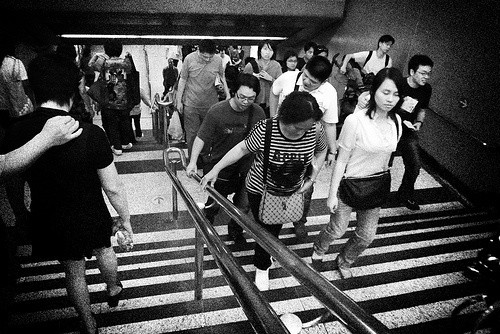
[
  {"xmin": 416, "ymin": 120, "xmax": 424, "ymax": 127},
  {"xmin": 330, "ymin": 151, "xmax": 338, "ymax": 156}
]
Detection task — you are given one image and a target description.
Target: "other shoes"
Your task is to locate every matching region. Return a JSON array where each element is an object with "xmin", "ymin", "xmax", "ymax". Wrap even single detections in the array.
[
  {"xmin": 397, "ymin": 196, "xmax": 420, "ymax": 211},
  {"xmin": 311, "ymin": 242, "xmax": 323, "ymax": 271},
  {"xmin": 121, "ymin": 143, "xmax": 132, "ymax": 150},
  {"xmin": 111, "ymin": 145, "xmax": 122, "ymax": 155},
  {"xmin": 294, "ymin": 222, "xmax": 309, "ymax": 242},
  {"xmin": 232, "ymin": 233, "xmax": 246, "ymax": 244},
  {"xmin": 106, "ymin": 278, "xmax": 123, "ymax": 307},
  {"xmin": 90, "ymin": 312, "xmax": 99, "ymax": 334},
  {"xmin": 206, "ymin": 214, "xmax": 214, "ymax": 226},
  {"xmin": 335, "ymin": 261, "xmax": 354, "ymax": 288},
  {"xmin": 269, "ymin": 256, "xmax": 277, "ymax": 269},
  {"xmin": 135, "ymin": 132, "xmax": 145, "ymax": 142},
  {"xmin": 254, "ymin": 267, "xmax": 270, "ymax": 291}
]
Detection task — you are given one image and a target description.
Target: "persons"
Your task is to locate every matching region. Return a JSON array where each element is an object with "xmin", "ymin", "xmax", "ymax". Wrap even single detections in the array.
[
  {"xmin": 0, "ymin": 115, "xmax": 84, "ymax": 316},
  {"xmin": 311, "ymin": 67, "xmax": 403, "ymax": 279},
  {"xmin": 199, "ymin": 90, "xmax": 330, "ymax": 293},
  {"xmin": 270, "ymin": 56, "xmax": 339, "ymax": 238},
  {"xmin": 187, "ymin": 73, "xmax": 268, "ymax": 248},
  {"xmin": 0, "ymin": 53, "xmax": 133, "ymax": 334},
  {"xmin": 0, "ymin": 33, "xmax": 392, "ymax": 224},
  {"xmin": 356, "ymin": 53, "xmax": 433, "ymax": 209}
]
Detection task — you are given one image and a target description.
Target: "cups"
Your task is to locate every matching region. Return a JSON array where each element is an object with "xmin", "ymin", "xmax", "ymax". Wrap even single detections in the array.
[{"xmin": 115, "ymin": 228, "xmax": 133, "ymax": 251}]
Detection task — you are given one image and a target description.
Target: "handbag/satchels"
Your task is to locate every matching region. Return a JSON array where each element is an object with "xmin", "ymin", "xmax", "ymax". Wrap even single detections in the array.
[
  {"xmin": 5, "ymin": 58, "xmax": 27, "ymax": 115},
  {"xmin": 338, "ymin": 170, "xmax": 391, "ymax": 210},
  {"xmin": 226, "ymin": 154, "xmax": 254, "ymax": 186},
  {"xmin": 167, "ymin": 111, "xmax": 183, "ymax": 140},
  {"xmin": 258, "ymin": 185, "xmax": 305, "ymax": 225},
  {"xmin": 87, "ymin": 58, "xmax": 110, "ymax": 110}
]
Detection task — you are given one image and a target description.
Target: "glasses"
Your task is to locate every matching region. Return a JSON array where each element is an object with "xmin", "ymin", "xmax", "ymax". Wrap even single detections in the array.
[
  {"xmin": 315, "ymin": 47, "xmax": 328, "ymax": 55},
  {"xmin": 236, "ymin": 91, "xmax": 255, "ymax": 101},
  {"xmin": 417, "ymin": 70, "xmax": 432, "ymax": 76}
]
